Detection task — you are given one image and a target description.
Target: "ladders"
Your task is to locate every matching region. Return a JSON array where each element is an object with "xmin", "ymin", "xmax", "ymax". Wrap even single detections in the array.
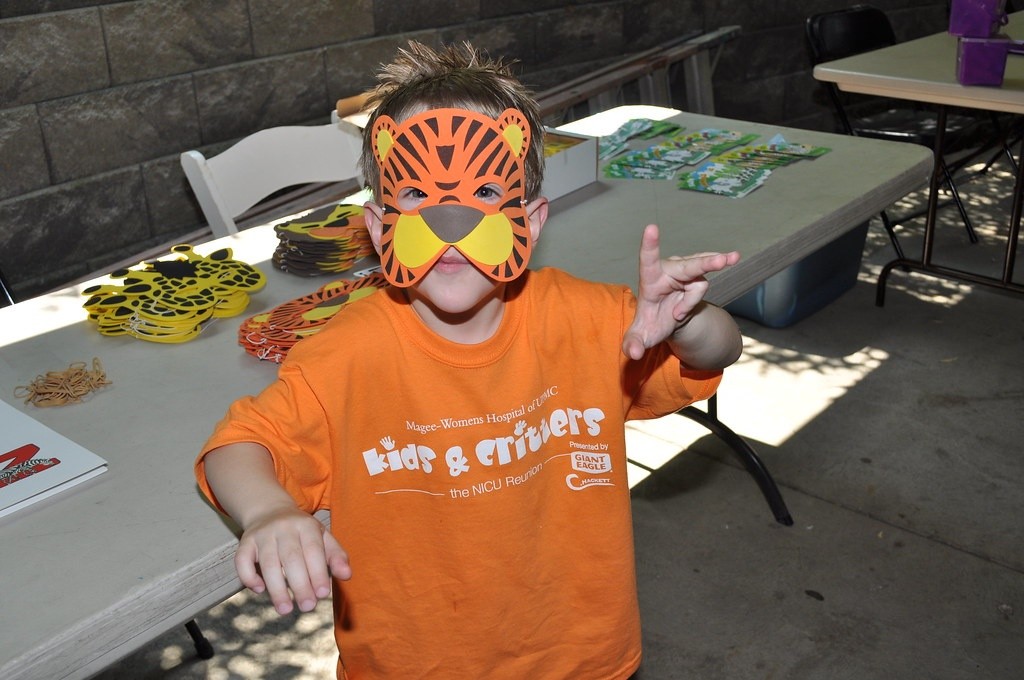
[{"xmin": 49, "ymin": 21, "xmax": 746, "ymax": 285}]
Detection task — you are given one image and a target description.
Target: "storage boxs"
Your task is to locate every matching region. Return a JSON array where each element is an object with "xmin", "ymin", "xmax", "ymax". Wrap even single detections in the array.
[{"xmin": 724, "ymin": 217, "xmax": 871, "ymax": 330}]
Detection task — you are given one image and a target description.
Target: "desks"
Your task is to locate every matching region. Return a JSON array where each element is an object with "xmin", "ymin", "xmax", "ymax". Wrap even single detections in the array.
[
  {"xmin": 0, "ymin": 104, "xmax": 937, "ymax": 680},
  {"xmin": 813, "ymin": 7, "xmax": 1024, "ymax": 308}
]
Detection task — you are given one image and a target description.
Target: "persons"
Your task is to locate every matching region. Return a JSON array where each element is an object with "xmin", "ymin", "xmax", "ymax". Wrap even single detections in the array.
[{"xmin": 193, "ymin": 38, "xmax": 743, "ymax": 680}]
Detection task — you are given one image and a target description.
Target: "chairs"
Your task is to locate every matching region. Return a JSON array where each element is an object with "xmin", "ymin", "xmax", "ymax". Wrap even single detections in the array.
[{"xmin": 179, "ymin": 107, "xmax": 373, "ymax": 239}]
